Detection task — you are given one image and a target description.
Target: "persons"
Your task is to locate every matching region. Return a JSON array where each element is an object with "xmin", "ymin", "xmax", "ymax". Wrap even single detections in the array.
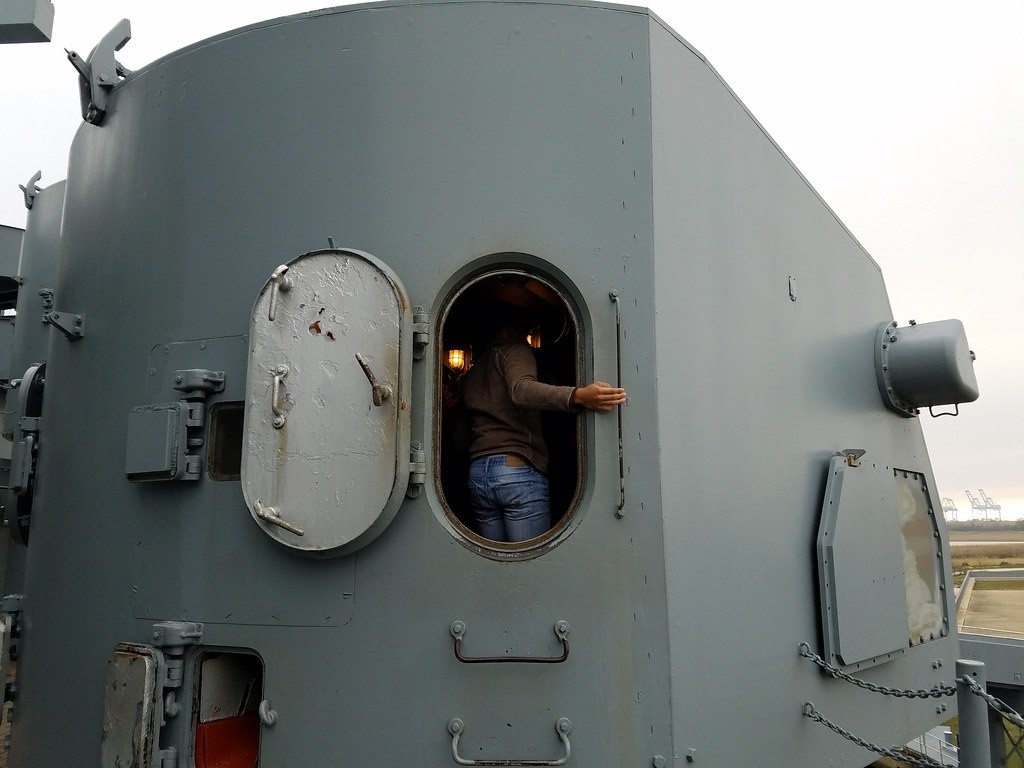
[{"xmin": 459, "ymin": 303, "xmax": 625, "ymax": 546}]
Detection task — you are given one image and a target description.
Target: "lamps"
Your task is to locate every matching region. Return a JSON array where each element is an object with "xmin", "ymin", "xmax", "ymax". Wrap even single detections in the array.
[{"xmin": 448, "ymin": 349, "xmax": 464, "ymax": 370}]
